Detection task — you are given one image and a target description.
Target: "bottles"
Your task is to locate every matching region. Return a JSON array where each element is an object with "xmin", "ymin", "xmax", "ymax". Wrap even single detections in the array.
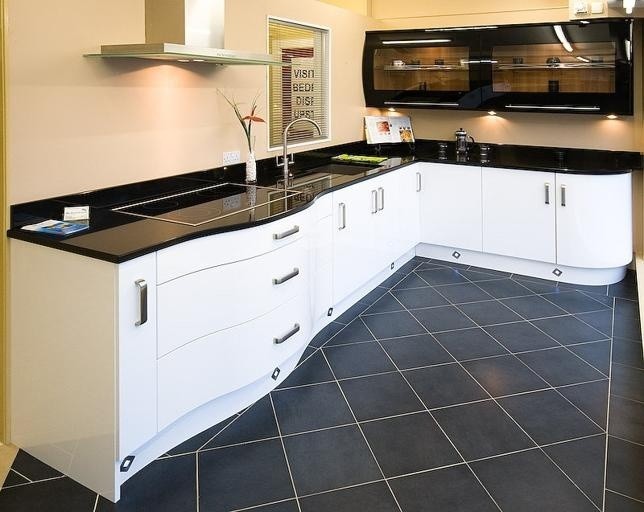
[
  {"xmin": 505, "ymin": 80, "xmax": 511, "ymax": 92},
  {"xmin": 460, "ymin": 57, "xmax": 468, "ymax": 66}
]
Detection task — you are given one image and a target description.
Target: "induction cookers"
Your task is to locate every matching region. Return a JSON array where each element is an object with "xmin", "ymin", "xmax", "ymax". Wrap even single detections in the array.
[{"xmin": 111, "ymin": 182, "xmax": 302, "ymax": 227}]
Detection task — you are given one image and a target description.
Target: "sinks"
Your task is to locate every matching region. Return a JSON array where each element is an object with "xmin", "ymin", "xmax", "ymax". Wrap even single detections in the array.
[{"xmin": 308, "ymin": 163, "xmax": 382, "ymax": 176}]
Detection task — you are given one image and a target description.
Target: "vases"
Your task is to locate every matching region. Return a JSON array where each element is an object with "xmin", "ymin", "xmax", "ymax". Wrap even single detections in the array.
[{"xmin": 246, "ymin": 152, "xmax": 257, "ymax": 183}]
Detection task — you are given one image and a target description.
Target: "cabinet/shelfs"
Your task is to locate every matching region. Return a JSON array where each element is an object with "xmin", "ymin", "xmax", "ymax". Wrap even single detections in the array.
[
  {"xmin": 331, "ymin": 159, "xmax": 421, "ymax": 321},
  {"xmin": 8, "ymin": 238, "xmax": 156, "ymax": 503},
  {"xmin": 481, "ymin": 16, "xmax": 636, "ymax": 115},
  {"xmin": 359, "ymin": 27, "xmax": 481, "ymax": 111},
  {"xmin": 157, "ymin": 193, "xmax": 331, "ymax": 459},
  {"xmin": 416, "ymin": 164, "xmax": 482, "ymax": 271},
  {"xmin": 482, "ymin": 166, "xmax": 632, "ymax": 286}
]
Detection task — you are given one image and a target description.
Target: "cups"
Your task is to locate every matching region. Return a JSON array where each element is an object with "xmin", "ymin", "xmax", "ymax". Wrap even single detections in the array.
[
  {"xmin": 411, "ymin": 60, "xmax": 420, "ymax": 68},
  {"xmin": 455, "ymin": 127, "xmax": 474, "ymax": 155},
  {"xmin": 592, "ymin": 56, "xmax": 603, "ymax": 67},
  {"xmin": 419, "ymin": 82, "xmax": 426, "ymax": 90},
  {"xmin": 456, "ymin": 156, "xmax": 470, "ymax": 164},
  {"xmin": 512, "ymin": 58, "xmax": 523, "ymax": 67},
  {"xmin": 435, "ymin": 59, "xmax": 444, "ymax": 67},
  {"xmin": 548, "ymin": 80, "xmax": 559, "ymax": 91},
  {"xmin": 393, "ymin": 59, "xmax": 405, "ymax": 69},
  {"xmin": 439, "ymin": 151, "xmax": 447, "ymax": 160},
  {"xmin": 438, "ymin": 143, "xmax": 448, "ymax": 151},
  {"xmin": 480, "ymin": 144, "xmax": 489, "ymax": 155}
]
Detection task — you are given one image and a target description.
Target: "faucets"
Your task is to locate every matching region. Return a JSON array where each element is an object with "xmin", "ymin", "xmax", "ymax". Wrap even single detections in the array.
[
  {"xmin": 284, "ymin": 180, "xmax": 288, "ymax": 209},
  {"xmin": 275, "ymin": 118, "xmax": 322, "ymax": 189}
]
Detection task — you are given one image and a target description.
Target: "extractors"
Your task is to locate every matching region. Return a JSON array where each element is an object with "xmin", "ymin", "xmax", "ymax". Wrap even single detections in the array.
[{"xmin": 83, "ymin": 1, "xmax": 283, "ymax": 67}]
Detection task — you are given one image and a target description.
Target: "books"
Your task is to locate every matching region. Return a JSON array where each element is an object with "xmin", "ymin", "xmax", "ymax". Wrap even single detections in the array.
[
  {"xmin": 364, "ymin": 115, "xmax": 415, "ymax": 144},
  {"xmin": 20, "ymin": 219, "xmax": 90, "ymax": 236},
  {"xmin": 330, "ymin": 153, "xmax": 388, "ymax": 165}
]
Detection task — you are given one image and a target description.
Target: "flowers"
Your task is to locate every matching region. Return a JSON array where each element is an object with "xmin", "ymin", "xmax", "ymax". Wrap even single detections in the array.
[{"xmin": 214, "ymin": 87, "xmax": 267, "ymax": 152}]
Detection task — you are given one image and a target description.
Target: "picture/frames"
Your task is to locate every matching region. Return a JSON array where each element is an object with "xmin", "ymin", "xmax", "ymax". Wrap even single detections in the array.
[{"xmin": 282, "ymin": 48, "xmax": 313, "ymax": 141}]
